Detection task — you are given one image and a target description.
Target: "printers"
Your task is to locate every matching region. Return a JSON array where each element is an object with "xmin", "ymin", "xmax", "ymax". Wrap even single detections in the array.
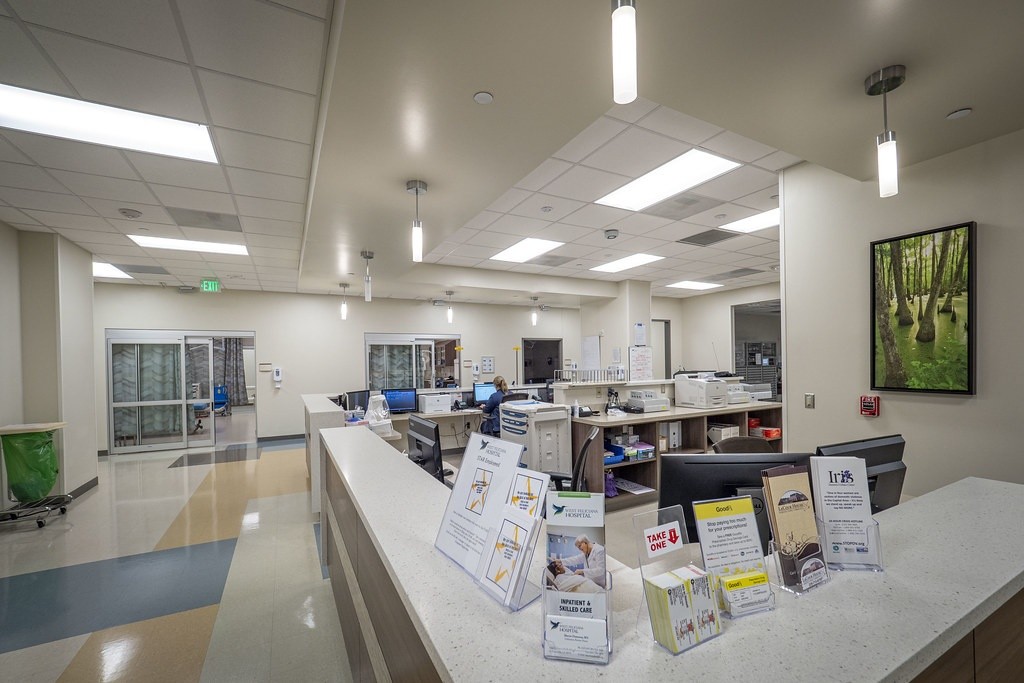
[
  {"xmin": 675, "ymin": 372, "xmax": 727, "ymax": 409},
  {"xmin": 419, "ymin": 394, "xmax": 452, "ymax": 415}
]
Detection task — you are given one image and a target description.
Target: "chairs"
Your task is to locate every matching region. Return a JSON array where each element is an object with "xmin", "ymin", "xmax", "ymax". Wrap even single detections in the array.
[
  {"xmin": 541, "ymin": 424, "xmax": 601, "ymax": 490},
  {"xmin": 713, "ymin": 437, "xmax": 777, "ymax": 454},
  {"xmin": 481, "ymin": 393, "xmax": 530, "ymax": 441}
]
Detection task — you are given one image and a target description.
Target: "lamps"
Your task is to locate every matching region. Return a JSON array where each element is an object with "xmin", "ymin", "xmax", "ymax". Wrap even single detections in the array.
[
  {"xmin": 864, "ymin": 65, "xmax": 905, "ymax": 199},
  {"xmin": 446, "ymin": 291, "xmax": 455, "ymax": 325},
  {"xmin": 611, "ymin": 0, "xmax": 637, "ymax": 105},
  {"xmin": 406, "ymin": 179, "xmax": 428, "ymax": 262},
  {"xmin": 361, "ymin": 249, "xmax": 375, "ymax": 302},
  {"xmin": 531, "ymin": 297, "xmax": 538, "ymax": 326},
  {"xmin": 339, "ymin": 282, "xmax": 350, "ymax": 322}
]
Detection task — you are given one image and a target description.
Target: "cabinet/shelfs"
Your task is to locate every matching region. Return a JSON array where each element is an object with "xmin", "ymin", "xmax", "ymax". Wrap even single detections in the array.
[{"xmin": 571, "ymin": 402, "xmax": 782, "ymax": 513}]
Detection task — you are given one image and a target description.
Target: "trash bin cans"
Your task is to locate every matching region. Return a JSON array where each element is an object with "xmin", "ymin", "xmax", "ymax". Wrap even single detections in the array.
[
  {"xmin": 214, "ymin": 386, "xmax": 228, "ymax": 415},
  {"xmin": 0, "ymin": 422, "xmax": 67, "ymax": 505}
]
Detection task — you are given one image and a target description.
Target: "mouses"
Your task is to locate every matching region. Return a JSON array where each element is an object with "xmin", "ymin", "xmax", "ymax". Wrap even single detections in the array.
[{"xmin": 443, "ymin": 468, "xmax": 454, "ymax": 476}]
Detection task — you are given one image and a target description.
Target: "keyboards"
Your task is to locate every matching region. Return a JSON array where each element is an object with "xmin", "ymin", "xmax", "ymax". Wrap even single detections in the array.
[{"xmin": 444, "ymin": 478, "xmax": 454, "ymax": 490}]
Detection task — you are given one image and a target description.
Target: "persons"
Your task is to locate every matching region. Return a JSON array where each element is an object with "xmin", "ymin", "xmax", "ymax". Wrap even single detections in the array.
[
  {"xmin": 479, "ymin": 376, "xmax": 514, "ymax": 434},
  {"xmin": 547, "ymin": 533, "xmax": 606, "ymax": 592}
]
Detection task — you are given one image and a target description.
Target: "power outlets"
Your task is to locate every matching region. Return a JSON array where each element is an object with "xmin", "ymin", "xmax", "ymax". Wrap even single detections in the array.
[{"xmin": 451, "ymin": 423, "xmax": 456, "ymax": 431}]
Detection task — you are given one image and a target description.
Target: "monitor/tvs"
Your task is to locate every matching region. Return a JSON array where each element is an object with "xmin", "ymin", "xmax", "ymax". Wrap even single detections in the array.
[
  {"xmin": 473, "ymin": 383, "xmax": 497, "ymax": 406},
  {"xmin": 346, "ymin": 389, "xmax": 370, "ymax": 414},
  {"xmin": 407, "ymin": 413, "xmax": 445, "ymax": 485},
  {"xmin": 381, "ymin": 388, "xmax": 417, "ymax": 414},
  {"xmin": 657, "ymin": 453, "xmax": 813, "ymax": 558},
  {"xmin": 814, "ymin": 433, "xmax": 907, "ymax": 514}
]
